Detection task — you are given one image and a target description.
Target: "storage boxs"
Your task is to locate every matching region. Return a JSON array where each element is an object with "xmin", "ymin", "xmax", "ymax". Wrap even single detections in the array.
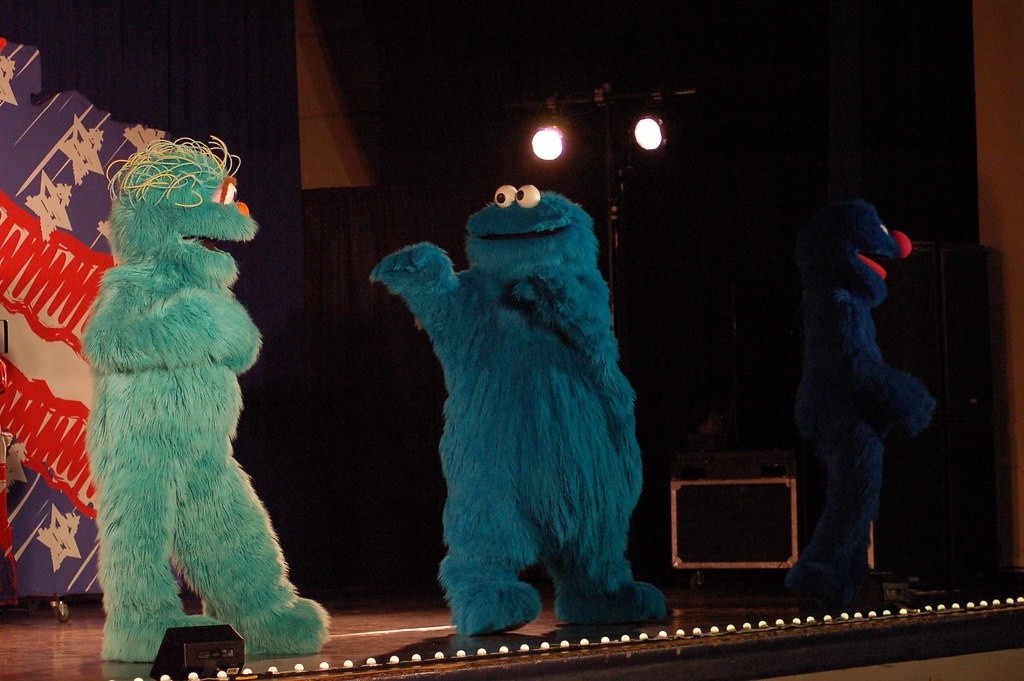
[{"xmin": 670, "ymin": 449, "xmax": 801, "ymax": 578}]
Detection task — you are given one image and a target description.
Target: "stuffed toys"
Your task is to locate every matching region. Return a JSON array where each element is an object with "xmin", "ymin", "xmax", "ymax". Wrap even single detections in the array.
[
  {"xmin": 772, "ymin": 196, "xmax": 939, "ymax": 611},
  {"xmin": 364, "ymin": 181, "xmax": 671, "ymax": 635},
  {"xmin": 79, "ymin": 139, "xmax": 329, "ymax": 661}
]
